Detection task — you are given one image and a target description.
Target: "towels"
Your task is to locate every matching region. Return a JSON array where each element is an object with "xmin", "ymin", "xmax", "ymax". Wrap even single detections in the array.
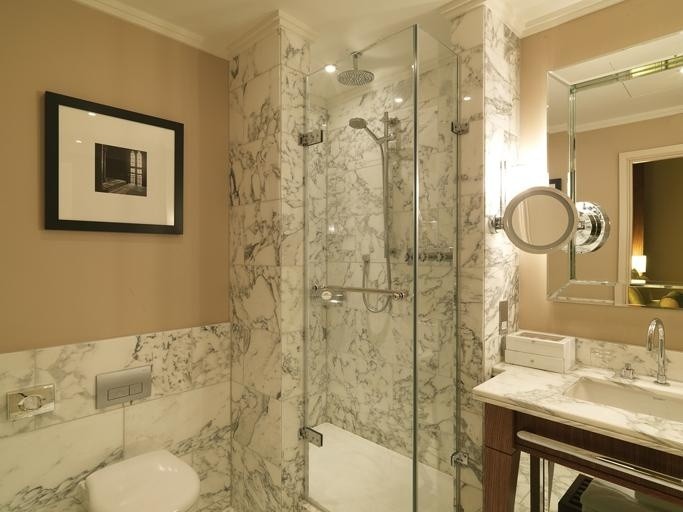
[{"xmin": 575, "ymin": 476, "xmax": 683, "ymax": 512}]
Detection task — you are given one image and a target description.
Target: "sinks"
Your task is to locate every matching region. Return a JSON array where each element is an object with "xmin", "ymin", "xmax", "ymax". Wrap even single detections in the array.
[{"xmin": 562, "ymin": 375, "xmax": 683, "ymax": 427}]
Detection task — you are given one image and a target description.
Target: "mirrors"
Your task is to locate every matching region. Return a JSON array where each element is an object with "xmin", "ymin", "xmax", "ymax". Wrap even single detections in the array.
[
  {"xmin": 544, "ymin": 29, "xmax": 682, "ymax": 310},
  {"xmin": 487, "ymin": 185, "xmax": 577, "ymax": 256},
  {"xmin": 508, "ymin": 449, "xmax": 683, "ymax": 512},
  {"xmin": 572, "ymin": 200, "xmax": 610, "ymax": 255}
]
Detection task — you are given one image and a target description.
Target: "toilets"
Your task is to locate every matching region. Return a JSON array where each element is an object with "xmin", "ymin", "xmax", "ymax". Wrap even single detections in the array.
[{"xmin": 76, "ymin": 448, "xmax": 202, "ymax": 512}]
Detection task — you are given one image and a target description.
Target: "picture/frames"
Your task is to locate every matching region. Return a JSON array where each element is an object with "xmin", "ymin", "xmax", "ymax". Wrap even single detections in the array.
[
  {"xmin": 548, "ymin": 178, "xmax": 562, "ymax": 193},
  {"xmin": 41, "ymin": 89, "xmax": 184, "ymax": 236}
]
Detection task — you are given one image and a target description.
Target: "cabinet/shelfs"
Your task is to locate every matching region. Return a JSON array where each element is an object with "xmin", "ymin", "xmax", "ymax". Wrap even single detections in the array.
[{"xmin": 479, "ymin": 402, "xmax": 682, "ymax": 512}]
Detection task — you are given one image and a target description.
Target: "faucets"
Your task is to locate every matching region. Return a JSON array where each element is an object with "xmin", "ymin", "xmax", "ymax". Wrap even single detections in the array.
[{"xmin": 645, "ymin": 316, "xmax": 668, "ymax": 386}]
